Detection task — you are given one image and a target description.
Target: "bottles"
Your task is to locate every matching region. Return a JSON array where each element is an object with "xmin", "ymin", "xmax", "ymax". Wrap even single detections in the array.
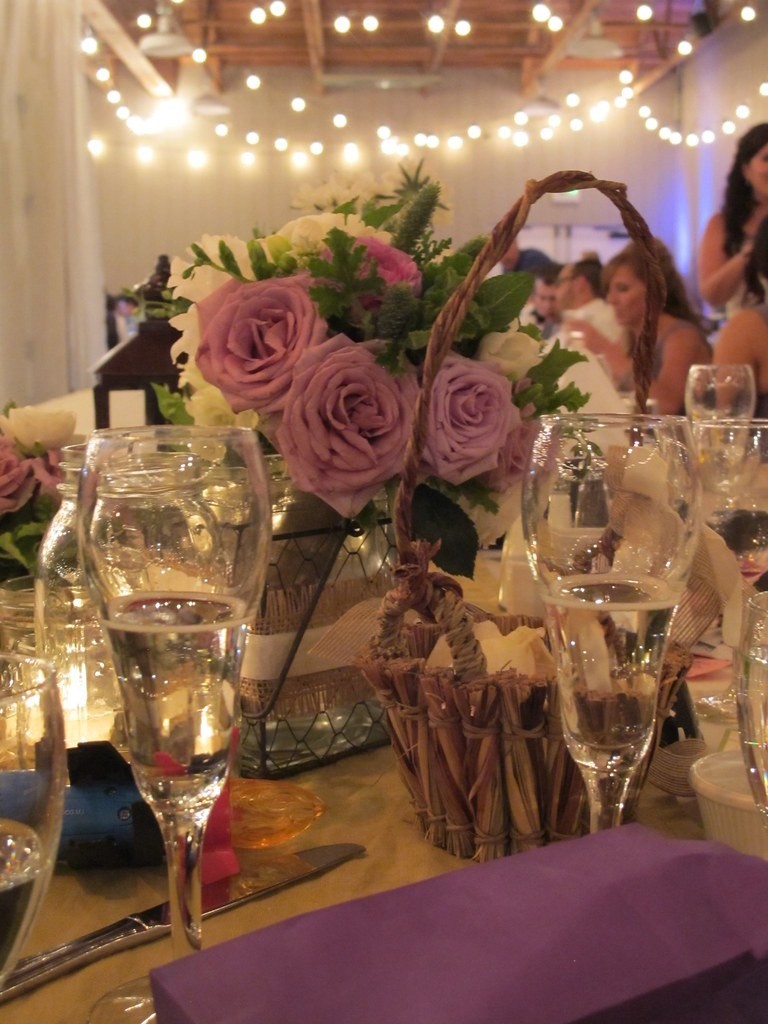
[{"xmin": 35, "ymin": 442, "xmax": 119, "ymax": 752}]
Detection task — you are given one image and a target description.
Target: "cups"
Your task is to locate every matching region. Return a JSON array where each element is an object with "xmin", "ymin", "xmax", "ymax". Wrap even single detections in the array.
[
  {"xmin": 0, "ymin": 575, "xmax": 42, "ymax": 656},
  {"xmin": 733, "ymin": 591, "xmax": 768, "ymax": 830},
  {"xmin": 0, "ymin": 652, "xmax": 67, "ymax": 979},
  {"xmin": 690, "ymin": 417, "xmax": 768, "ymax": 586},
  {"xmin": 684, "ymin": 363, "xmax": 757, "ymax": 467}
]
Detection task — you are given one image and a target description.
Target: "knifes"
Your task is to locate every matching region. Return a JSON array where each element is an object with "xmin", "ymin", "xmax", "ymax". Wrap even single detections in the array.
[{"xmin": 0, "ymin": 844, "xmax": 367, "ymax": 1003}]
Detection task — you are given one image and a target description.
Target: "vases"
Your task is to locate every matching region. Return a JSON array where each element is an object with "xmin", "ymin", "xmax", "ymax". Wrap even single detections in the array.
[{"xmin": 232, "ymin": 451, "xmax": 398, "ymax": 781}]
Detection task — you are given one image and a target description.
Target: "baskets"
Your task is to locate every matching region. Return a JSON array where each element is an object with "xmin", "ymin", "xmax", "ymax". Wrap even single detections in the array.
[{"xmin": 359, "ymin": 171, "xmax": 692, "ymax": 855}]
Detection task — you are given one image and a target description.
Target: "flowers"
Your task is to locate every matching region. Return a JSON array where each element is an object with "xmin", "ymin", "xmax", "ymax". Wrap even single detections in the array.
[
  {"xmin": 0, "ymin": 399, "xmax": 77, "ymax": 593},
  {"xmin": 123, "ymin": 157, "xmax": 592, "ymax": 581}
]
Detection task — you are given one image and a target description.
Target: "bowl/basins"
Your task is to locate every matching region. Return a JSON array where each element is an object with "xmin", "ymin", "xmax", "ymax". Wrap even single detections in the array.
[{"xmin": 687, "ymin": 749, "xmax": 768, "ymax": 861}]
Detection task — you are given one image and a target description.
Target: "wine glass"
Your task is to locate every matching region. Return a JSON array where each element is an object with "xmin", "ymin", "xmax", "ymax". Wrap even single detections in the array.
[
  {"xmin": 520, "ymin": 412, "xmax": 701, "ymax": 836},
  {"xmin": 76, "ymin": 423, "xmax": 274, "ymax": 1024}
]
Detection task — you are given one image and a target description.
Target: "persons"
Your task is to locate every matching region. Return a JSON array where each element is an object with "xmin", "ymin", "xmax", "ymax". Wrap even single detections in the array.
[
  {"xmin": 701, "ymin": 121, "xmax": 768, "ymax": 320},
  {"xmin": 501, "ymin": 240, "xmax": 627, "ymax": 389},
  {"xmin": 568, "ymin": 239, "xmax": 714, "ymax": 441},
  {"xmin": 712, "ymin": 214, "xmax": 768, "ymax": 463}
]
